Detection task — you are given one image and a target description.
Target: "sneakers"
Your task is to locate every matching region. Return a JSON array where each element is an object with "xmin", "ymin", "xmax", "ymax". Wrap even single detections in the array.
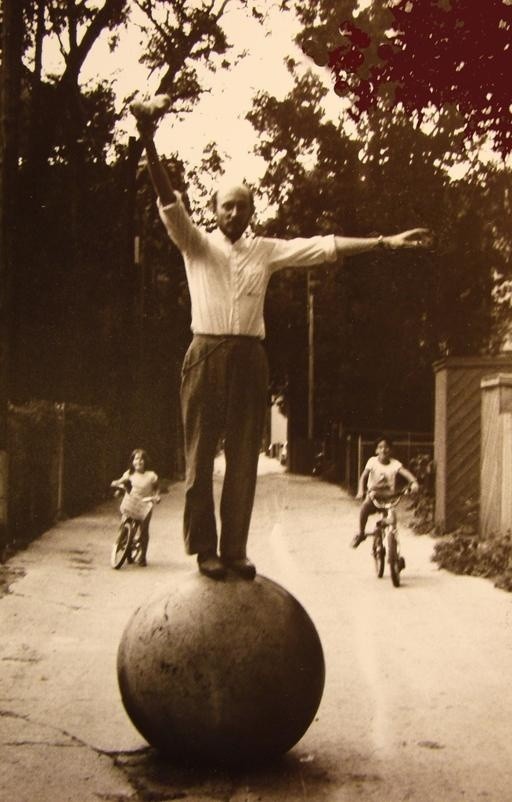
[
  {"xmin": 139, "ymin": 556, "xmax": 147, "ymax": 566},
  {"xmin": 352, "ymin": 534, "xmax": 365, "ymax": 547},
  {"xmin": 197, "ymin": 555, "xmax": 225, "ymax": 578},
  {"xmin": 220, "ymin": 553, "xmax": 255, "ymax": 575}
]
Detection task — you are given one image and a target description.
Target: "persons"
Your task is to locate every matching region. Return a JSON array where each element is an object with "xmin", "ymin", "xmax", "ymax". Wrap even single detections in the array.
[
  {"xmin": 110, "ymin": 450, "xmax": 163, "ymax": 567},
  {"xmin": 348, "ymin": 436, "xmax": 421, "ymax": 571},
  {"xmin": 136, "ymin": 111, "xmax": 436, "ymax": 579}
]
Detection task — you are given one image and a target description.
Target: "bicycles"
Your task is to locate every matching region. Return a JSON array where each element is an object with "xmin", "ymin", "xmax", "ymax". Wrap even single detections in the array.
[
  {"xmin": 110, "ymin": 482, "xmax": 159, "ymax": 569},
  {"xmin": 351, "ymin": 480, "xmax": 412, "ymax": 588}
]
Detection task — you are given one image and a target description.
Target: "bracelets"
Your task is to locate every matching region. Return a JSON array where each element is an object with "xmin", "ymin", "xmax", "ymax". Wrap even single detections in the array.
[{"xmin": 377, "ymin": 232, "xmax": 390, "ymax": 254}]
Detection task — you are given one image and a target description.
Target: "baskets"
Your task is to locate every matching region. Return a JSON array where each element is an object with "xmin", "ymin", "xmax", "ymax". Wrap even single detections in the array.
[{"xmin": 120, "ymin": 493, "xmax": 152, "ymax": 521}]
